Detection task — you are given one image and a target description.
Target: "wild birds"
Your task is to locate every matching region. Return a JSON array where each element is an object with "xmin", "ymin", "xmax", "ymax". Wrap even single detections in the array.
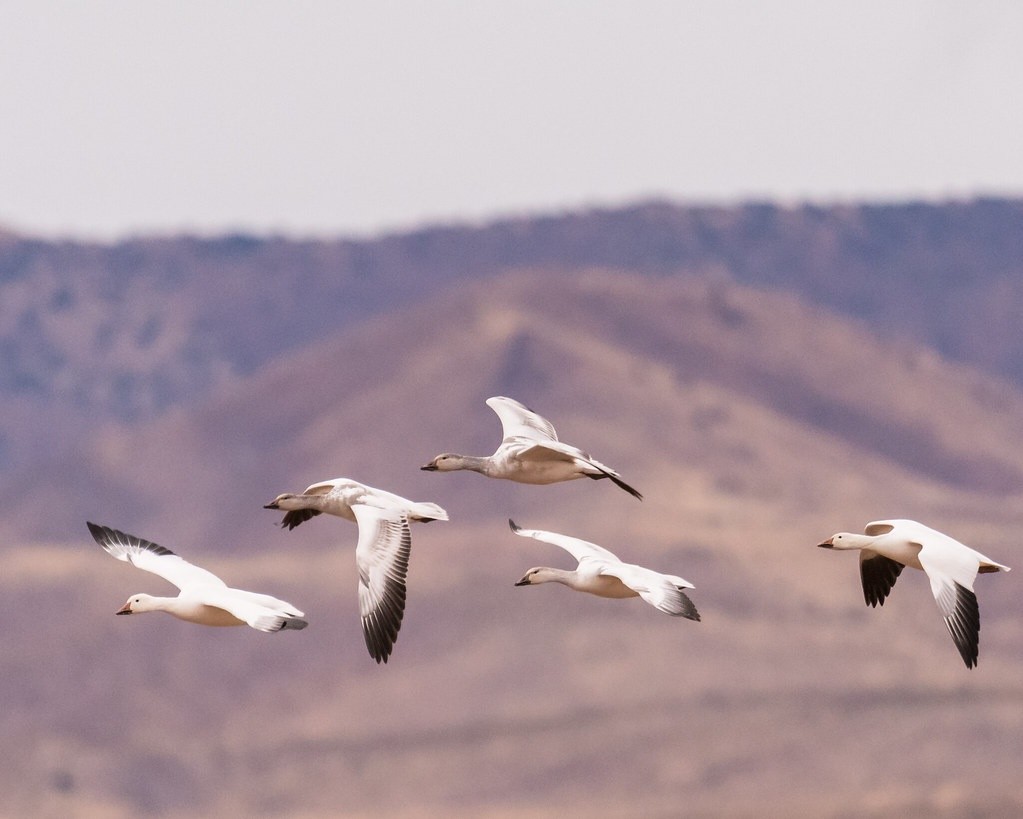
[
  {"xmin": 263, "ymin": 478, "xmax": 449, "ymax": 665},
  {"xmin": 87, "ymin": 522, "xmax": 308, "ymax": 635},
  {"xmin": 816, "ymin": 518, "xmax": 1012, "ymax": 670},
  {"xmin": 420, "ymin": 396, "xmax": 644, "ymax": 501},
  {"xmin": 509, "ymin": 518, "xmax": 701, "ymax": 622}
]
